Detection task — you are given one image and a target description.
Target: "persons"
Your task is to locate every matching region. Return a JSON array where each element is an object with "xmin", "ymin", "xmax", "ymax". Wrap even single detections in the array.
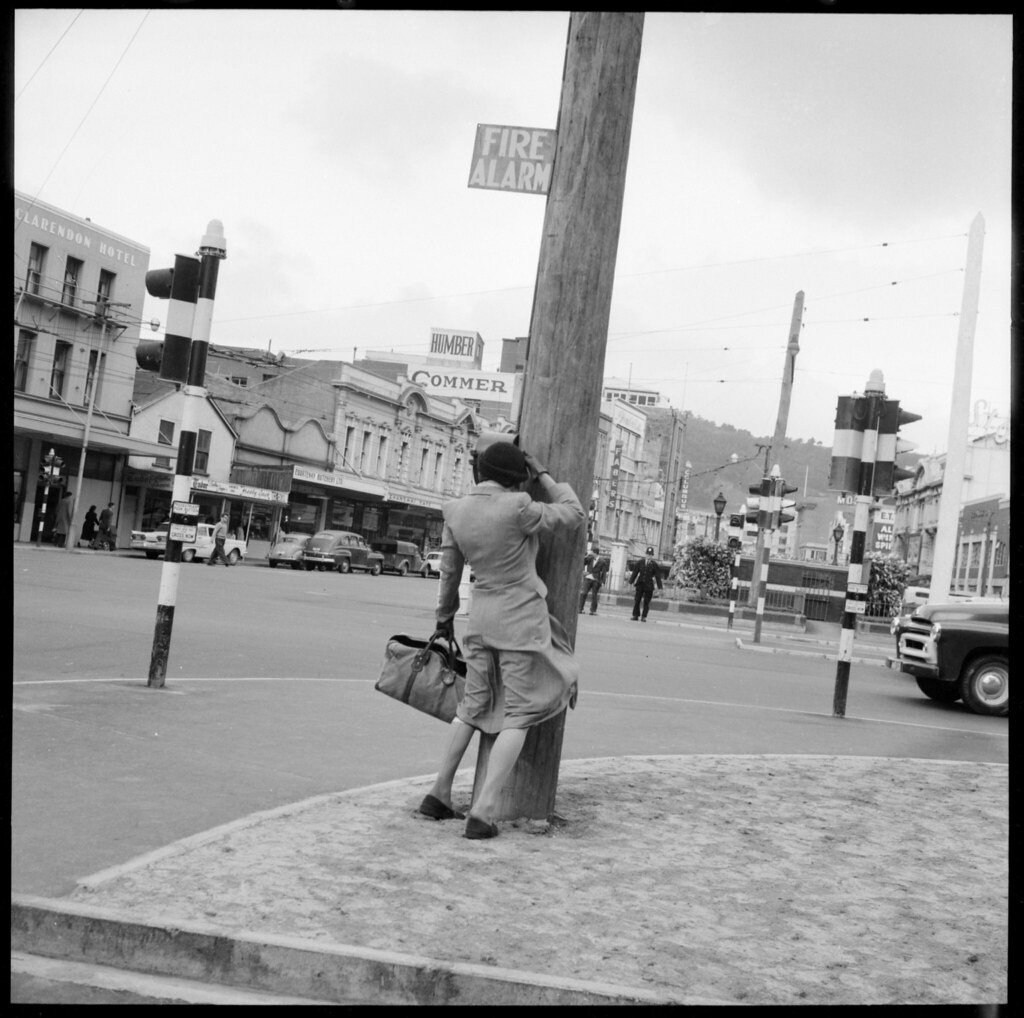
[
  {"xmin": 207, "ymin": 514, "xmax": 230, "ymax": 566},
  {"xmin": 418, "ymin": 442, "xmax": 587, "ymax": 840},
  {"xmin": 78, "ymin": 505, "xmax": 99, "ymax": 548},
  {"xmin": 53, "ymin": 491, "xmax": 73, "ymax": 548},
  {"xmin": 629, "ymin": 547, "xmax": 663, "ymax": 622},
  {"xmin": 93, "ymin": 501, "xmax": 117, "ymax": 551},
  {"xmin": 578, "ymin": 547, "xmax": 608, "ymax": 615}
]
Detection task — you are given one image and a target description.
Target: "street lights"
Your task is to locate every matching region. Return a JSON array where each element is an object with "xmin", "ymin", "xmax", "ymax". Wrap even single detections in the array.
[
  {"xmin": 831, "ymin": 525, "xmax": 844, "ymax": 568},
  {"xmin": 712, "ymin": 492, "xmax": 727, "ymax": 542}
]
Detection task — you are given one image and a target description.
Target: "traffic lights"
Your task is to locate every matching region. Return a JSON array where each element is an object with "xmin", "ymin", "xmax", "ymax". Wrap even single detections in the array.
[
  {"xmin": 871, "ymin": 399, "xmax": 923, "ymax": 496},
  {"xmin": 730, "ymin": 513, "xmax": 743, "ymax": 527},
  {"xmin": 771, "ymin": 480, "xmax": 798, "ymax": 530},
  {"xmin": 726, "ymin": 535, "xmax": 740, "ymax": 551},
  {"xmin": 745, "ymin": 478, "xmax": 762, "ymax": 523},
  {"xmin": 50, "ymin": 456, "xmax": 66, "ymax": 487},
  {"xmin": 37, "ymin": 456, "xmax": 53, "ymax": 484},
  {"xmin": 135, "ymin": 252, "xmax": 202, "ymax": 384}
]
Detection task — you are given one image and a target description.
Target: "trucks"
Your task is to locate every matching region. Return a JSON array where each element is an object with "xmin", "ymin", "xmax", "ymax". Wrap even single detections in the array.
[{"xmin": 369, "ymin": 537, "xmax": 425, "ymax": 576}]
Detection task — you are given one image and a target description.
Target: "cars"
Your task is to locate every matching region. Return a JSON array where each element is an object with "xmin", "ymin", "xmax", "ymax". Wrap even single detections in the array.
[
  {"xmin": 299, "ymin": 529, "xmax": 386, "ymax": 577},
  {"xmin": 420, "ymin": 551, "xmax": 444, "ymax": 579},
  {"xmin": 131, "ymin": 520, "xmax": 248, "ymax": 566},
  {"xmin": 267, "ymin": 532, "xmax": 313, "ymax": 570}
]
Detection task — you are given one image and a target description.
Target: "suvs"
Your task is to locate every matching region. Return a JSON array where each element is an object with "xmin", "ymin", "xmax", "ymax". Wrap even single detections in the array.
[{"xmin": 882, "ymin": 598, "xmax": 1009, "ymax": 717}]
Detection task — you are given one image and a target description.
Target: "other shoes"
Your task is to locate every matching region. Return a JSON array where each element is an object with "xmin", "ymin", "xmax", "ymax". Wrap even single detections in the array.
[
  {"xmin": 226, "ymin": 563, "xmax": 234, "ymax": 567},
  {"xmin": 58, "ymin": 544, "xmax": 65, "ymax": 547},
  {"xmin": 464, "ymin": 816, "xmax": 498, "ymax": 840},
  {"xmin": 110, "ymin": 546, "xmax": 116, "ymax": 551},
  {"xmin": 88, "ymin": 545, "xmax": 93, "ymax": 548},
  {"xmin": 78, "ymin": 542, "xmax": 81, "ymax": 547},
  {"xmin": 94, "ymin": 547, "xmax": 98, "ymax": 550},
  {"xmin": 207, "ymin": 561, "xmax": 216, "ymax": 565},
  {"xmin": 420, "ymin": 796, "xmax": 466, "ymax": 820},
  {"xmin": 631, "ymin": 616, "xmax": 638, "ymax": 620},
  {"xmin": 641, "ymin": 616, "xmax": 646, "ymax": 622}
]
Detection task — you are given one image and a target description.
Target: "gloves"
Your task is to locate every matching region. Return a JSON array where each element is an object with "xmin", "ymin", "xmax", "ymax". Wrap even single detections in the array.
[
  {"xmin": 521, "ymin": 450, "xmax": 550, "ymax": 483},
  {"xmin": 436, "ymin": 616, "xmax": 454, "ymax": 642}
]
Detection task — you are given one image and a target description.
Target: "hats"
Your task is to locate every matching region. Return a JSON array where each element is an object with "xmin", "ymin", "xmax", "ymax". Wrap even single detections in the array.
[
  {"xmin": 646, "ymin": 547, "xmax": 654, "ymax": 556},
  {"xmin": 478, "ymin": 442, "xmax": 529, "ymax": 485}
]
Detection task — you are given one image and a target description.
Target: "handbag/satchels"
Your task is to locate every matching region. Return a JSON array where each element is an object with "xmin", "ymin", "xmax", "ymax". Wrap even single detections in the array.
[{"xmin": 376, "ymin": 629, "xmax": 467, "ymax": 723}]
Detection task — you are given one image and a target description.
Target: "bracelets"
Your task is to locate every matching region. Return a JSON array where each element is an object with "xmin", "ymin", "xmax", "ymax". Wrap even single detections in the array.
[{"xmin": 535, "ymin": 470, "xmax": 550, "ymax": 483}]
